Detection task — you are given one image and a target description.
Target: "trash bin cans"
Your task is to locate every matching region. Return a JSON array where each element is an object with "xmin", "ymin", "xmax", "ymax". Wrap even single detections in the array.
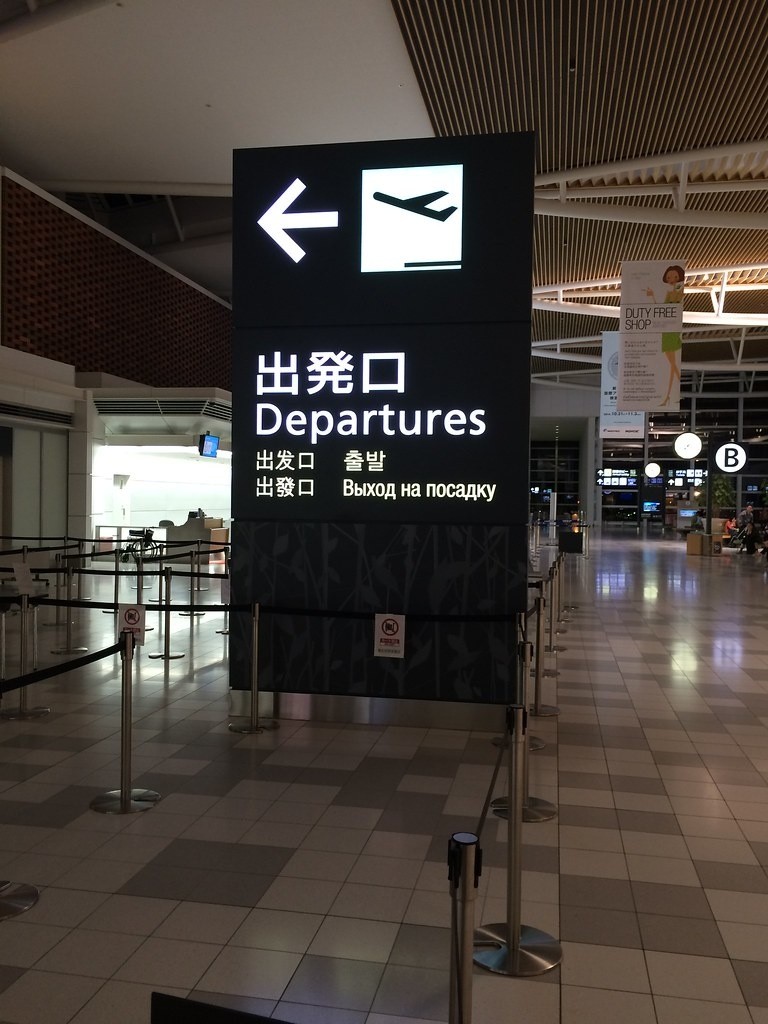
[{"xmin": 687, "ymin": 529, "xmax": 713, "ymax": 556}]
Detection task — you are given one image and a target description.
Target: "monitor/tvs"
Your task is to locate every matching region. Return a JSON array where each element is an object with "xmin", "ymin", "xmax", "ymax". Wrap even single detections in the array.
[{"xmin": 201, "ymin": 434, "xmax": 220, "ymax": 457}]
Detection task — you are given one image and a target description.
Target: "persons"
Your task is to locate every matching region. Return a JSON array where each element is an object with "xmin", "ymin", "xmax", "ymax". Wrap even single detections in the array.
[
  {"xmin": 723, "ymin": 503, "xmax": 768, "ymax": 569},
  {"xmin": 688, "ymin": 507, "xmax": 712, "ymax": 531}
]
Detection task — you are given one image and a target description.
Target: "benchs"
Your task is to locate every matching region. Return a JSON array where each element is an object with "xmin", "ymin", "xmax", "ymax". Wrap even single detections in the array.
[{"xmin": 700, "ymin": 518, "xmax": 736, "ymax": 546}]
